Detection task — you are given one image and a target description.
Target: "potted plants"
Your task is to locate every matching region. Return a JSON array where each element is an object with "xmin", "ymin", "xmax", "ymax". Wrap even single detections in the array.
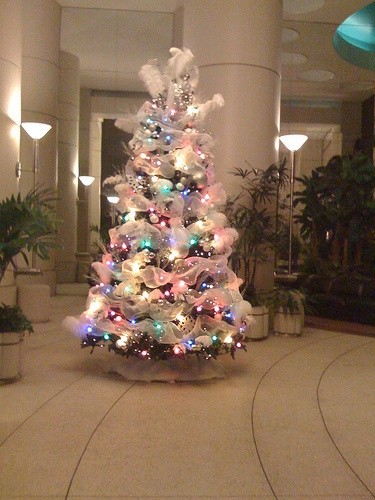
[
  {"xmin": 217, "ymin": 156, "xmax": 290, "ymax": 340},
  {"xmin": 0, "ymin": 182, "xmax": 62, "ymax": 384},
  {"xmin": 265, "ymin": 285, "xmax": 319, "ymax": 338}
]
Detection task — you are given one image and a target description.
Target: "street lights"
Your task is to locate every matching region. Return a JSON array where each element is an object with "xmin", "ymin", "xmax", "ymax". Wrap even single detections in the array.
[
  {"xmin": 75, "ymin": 174, "xmax": 95, "ymax": 204},
  {"xmin": 106, "ymin": 195, "xmax": 120, "ymax": 228},
  {"xmin": 14, "ymin": 121, "xmax": 53, "ymax": 275},
  {"xmin": 278, "ymin": 134, "xmax": 309, "ymax": 274}
]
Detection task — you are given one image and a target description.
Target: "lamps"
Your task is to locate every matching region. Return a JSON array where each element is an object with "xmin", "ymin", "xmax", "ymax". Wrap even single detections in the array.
[
  {"xmin": 279, "ymin": 134, "xmax": 308, "ymax": 152},
  {"xmin": 107, "ymin": 197, "xmax": 119, "ymax": 203},
  {"xmin": 79, "ymin": 175, "xmax": 94, "ymax": 186},
  {"xmin": 22, "ymin": 122, "xmax": 52, "ymax": 140}
]
